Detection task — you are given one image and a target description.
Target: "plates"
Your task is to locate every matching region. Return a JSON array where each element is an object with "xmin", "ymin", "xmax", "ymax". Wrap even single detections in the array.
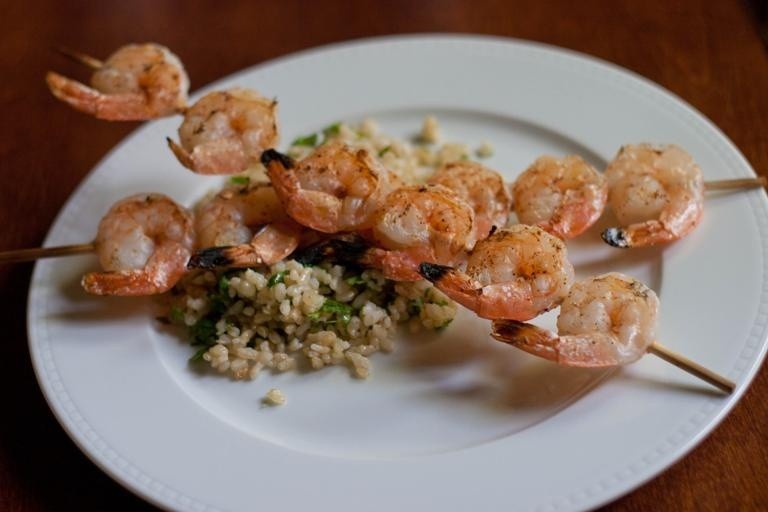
[{"xmin": 26, "ymin": 32, "xmax": 766, "ymax": 511}]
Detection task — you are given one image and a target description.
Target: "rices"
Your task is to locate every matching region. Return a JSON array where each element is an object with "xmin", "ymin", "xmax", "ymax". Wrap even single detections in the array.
[{"xmin": 172, "ymin": 116, "xmax": 493, "ymax": 406}]
